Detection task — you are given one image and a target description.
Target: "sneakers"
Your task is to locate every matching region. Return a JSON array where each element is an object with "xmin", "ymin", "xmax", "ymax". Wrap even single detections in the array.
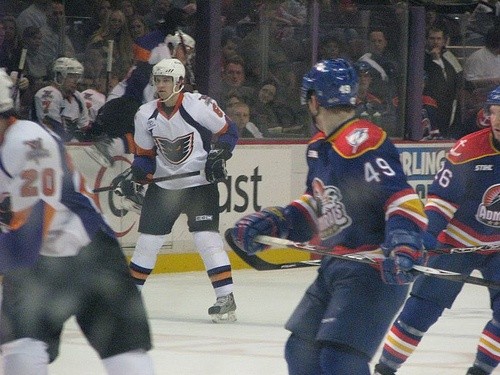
[{"xmin": 208, "ymin": 294, "xmax": 237, "ymax": 324}]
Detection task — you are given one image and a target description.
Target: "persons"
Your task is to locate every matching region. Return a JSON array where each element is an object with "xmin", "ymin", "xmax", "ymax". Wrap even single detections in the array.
[
  {"xmin": 421, "ymin": 21, "xmax": 500, "ymax": 140},
  {"xmin": 222, "ymin": 0, "xmax": 399, "ymax": 141},
  {"xmin": 0, "ymin": 68, "xmax": 153, "ymax": 375},
  {"xmin": 0, "ymin": 0, "xmax": 195, "ymax": 143},
  {"xmin": 233, "ymin": 59, "xmax": 429, "ymax": 375},
  {"xmin": 121, "ymin": 58, "xmax": 239, "ymax": 324},
  {"xmin": 373, "ymin": 85, "xmax": 500, "ymax": 375}
]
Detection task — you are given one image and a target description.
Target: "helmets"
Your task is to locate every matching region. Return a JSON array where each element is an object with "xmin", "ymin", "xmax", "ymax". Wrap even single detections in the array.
[
  {"xmin": 0, "ymin": 69, "xmax": 15, "ymax": 113},
  {"xmin": 165, "ymin": 31, "xmax": 195, "ymax": 49},
  {"xmin": 301, "ymin": 57, "xmax": 360, "ymax": 109},
  {"xmin": 486, "ymin": 86, "xmax": 500, "ymax": 105},
  {"xmin": 152, "ymin": 58, "xmax": 186, "ymax": 84},
  {"xmin": 52, "ymin": 57, "xmax": 84, "ymax": 74}
]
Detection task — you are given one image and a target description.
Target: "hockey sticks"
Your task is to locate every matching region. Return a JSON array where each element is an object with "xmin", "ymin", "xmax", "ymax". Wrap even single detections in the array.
[
  {"xmin": 224, "ymin": 228, "xmax": 500, "ymax": 271},
  {"xmin": 176, "ymin": 26, "xmax": 200, "ymax": 93},
  {"xmin": 10, "ymin": 46, "xmax": 29, "ymax": 109},
  {"xmin": 256, "ymin": 232, "xmax": 500, "ymax": 292},
  {"xmin": 79, "ymin": 166, "xmax": 205, "ymax": 194},
  {"xmin": 104, "ymin": 39, "xmax": 115, "ymax": 136}
]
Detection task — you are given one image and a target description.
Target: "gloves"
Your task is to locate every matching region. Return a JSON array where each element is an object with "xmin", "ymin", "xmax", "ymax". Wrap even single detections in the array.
[
  {"xmin": 232, "ymin": 206, "xmax": 290, "ymax": 256},
  {"xmin": 205, "ymin": 146, "xmax": 232, "ymax": 182},
  {"xmin": 422, "ymin": 231, "xmax": 438, "ymax": 256},
  {"xmin": 379, "ymin": 215, "xmax": 425, "ymax": 285},
  {"xmin": 130, "ymin": 155, "xmax": 155, "ymax": 186}
]
